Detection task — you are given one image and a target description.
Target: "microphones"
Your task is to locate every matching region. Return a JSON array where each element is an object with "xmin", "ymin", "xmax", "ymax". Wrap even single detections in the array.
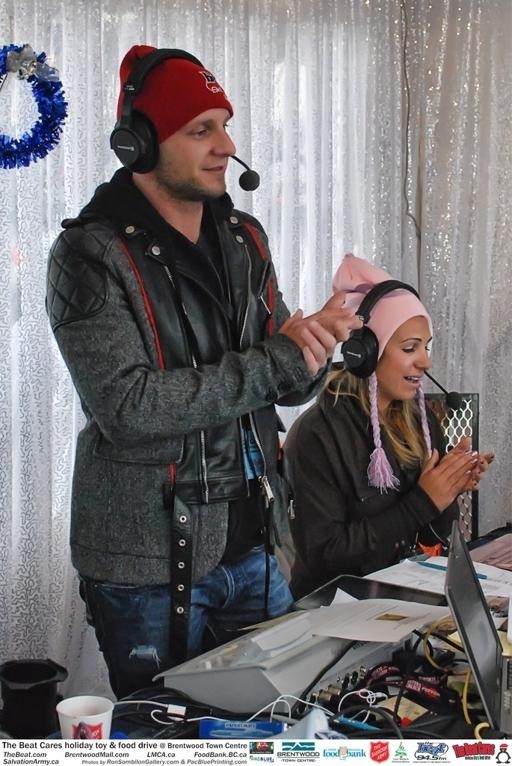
[
  {"xmin": 229, "ymin": 155, "xmax": 262, "ymax": 190},
  {"xmin": 425, "ymin": 371, "xmax": 462, "ymax": 409}
]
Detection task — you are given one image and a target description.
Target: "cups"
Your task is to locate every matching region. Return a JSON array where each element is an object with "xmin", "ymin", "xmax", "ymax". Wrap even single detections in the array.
[{"xmin": 56, "ymin": 695, "xmax": 114, "ymax": 740}]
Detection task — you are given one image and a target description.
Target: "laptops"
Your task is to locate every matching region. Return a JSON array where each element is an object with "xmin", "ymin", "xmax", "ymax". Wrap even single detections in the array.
[{"xmin": 444, "ymin": 518, "xmax": 511, "ymax": 734}]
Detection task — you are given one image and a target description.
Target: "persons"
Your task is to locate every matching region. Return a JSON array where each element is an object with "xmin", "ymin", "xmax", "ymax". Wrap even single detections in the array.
[
  {"xmin": 44, "ymin": 42, "xmax": 364, "ymax": 696},
  {"xmin": 282, "ymin": 253, "xmax": 495, "ymax": 600}
]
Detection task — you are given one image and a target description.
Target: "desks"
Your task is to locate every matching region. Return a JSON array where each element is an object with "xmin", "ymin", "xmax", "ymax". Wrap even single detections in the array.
[{"xmin": 48, "ymin": 516, "xmax": 512, "ymax": 738}]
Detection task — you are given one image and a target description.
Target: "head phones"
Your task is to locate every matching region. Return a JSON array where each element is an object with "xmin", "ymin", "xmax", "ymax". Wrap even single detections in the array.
[
  {"xmin": 109, "ymin": 48, "xmax": 202, "ymax": 174},
  {"xmin": 343, "ymin": 280, "xmax": 419, "ymax": 378}
]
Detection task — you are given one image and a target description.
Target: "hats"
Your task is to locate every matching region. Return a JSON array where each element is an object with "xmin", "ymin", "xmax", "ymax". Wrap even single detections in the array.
[
  {"xmin": 331, "ymin": 252, "xmax": 436, "ymax": 492},
  {"xmin": 115, "ymin": 43, "xmax": 232, "ymax": 141}
]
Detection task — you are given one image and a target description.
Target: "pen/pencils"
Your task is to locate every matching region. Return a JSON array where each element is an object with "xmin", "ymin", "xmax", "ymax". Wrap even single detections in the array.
[{"xmin": 416, "ymin": 561, "xmax": 487, "ymax": 579}]
